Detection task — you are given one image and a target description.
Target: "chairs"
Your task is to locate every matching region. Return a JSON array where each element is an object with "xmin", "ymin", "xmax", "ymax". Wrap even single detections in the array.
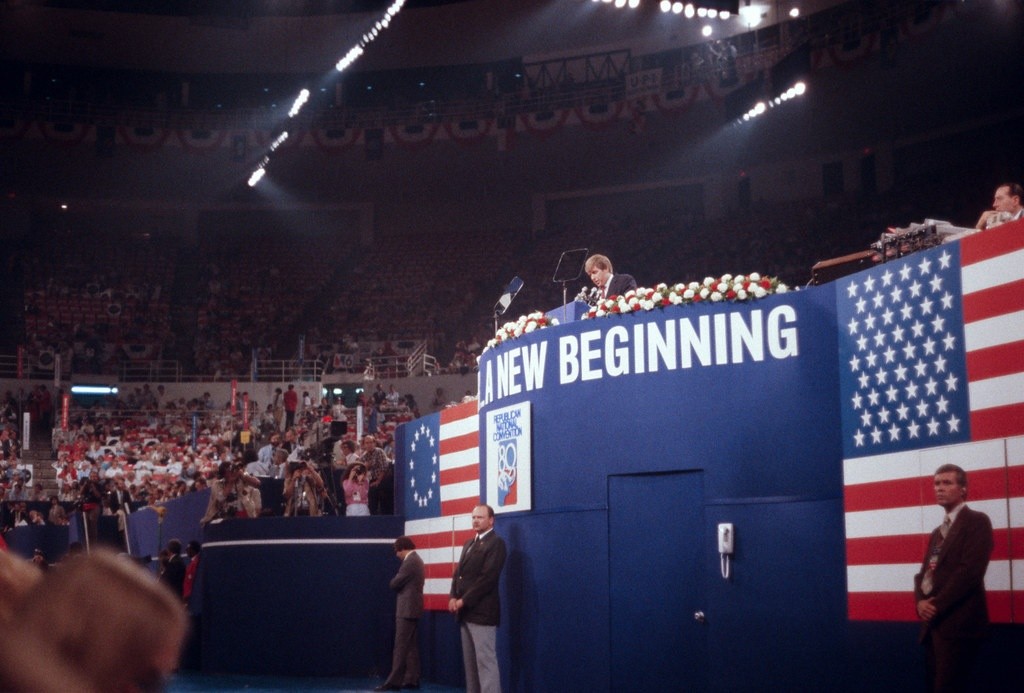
[{"xmin": 0, "ymin": 331, "xmax": 420, "ymax": 535}]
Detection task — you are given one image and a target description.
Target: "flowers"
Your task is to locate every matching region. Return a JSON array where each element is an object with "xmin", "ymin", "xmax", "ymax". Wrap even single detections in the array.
[
  {"xmin": 476, "ymin": 310, "xmax": 560, "ymax": 362},
  {"xmin": 582, "ymin": 269, "xmax": 789, "ymax": 320}
]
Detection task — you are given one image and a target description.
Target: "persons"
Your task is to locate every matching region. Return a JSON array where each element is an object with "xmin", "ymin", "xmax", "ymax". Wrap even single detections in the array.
[
  {"xmin": 374, "ymin": 535, "xmax": 424, "ymax": 692},
  {"xmin": 448, "ymin": 505, "xmax": 506, "ymax": 693},
  {"xmin": 916, "ymin": 468, "xmax": 992, "ymax": 693},
  {"xmin": 0, "ymin": 385, "xmax": 473, "ymax": 527},
  {"xmin": 976, "ymin": 182, "xmax": 1024, "ymax": 230},
  {"xmin": 157, "ymin": 539, "xmax": 201, "ymax": 608},
  {"xmin": 574, "ymin": 254, "xmax": 637, "ymax": 306},
  {"xmin": 31, "ymin": 548, "xmax": 48, "ymax": 577},
  {"xmin": 454, "ymin": 336, "xmax": 482, "ymax": 371},
  {"xmin": 66, "ymin": 541, "xmax": 82, "ymax": 561}
]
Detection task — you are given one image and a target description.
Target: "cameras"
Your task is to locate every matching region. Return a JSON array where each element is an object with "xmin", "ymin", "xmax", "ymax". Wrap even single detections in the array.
[
  {"xmin": 288, "ymin": 461, "xmax": 306, "ymax": 474},
  {"xmin": 356, "ymin": 465, "xmax": 366, "ymax": 475},
  {"xmin": 229, "ymin": 462, "xmax": 243, "ymax": 473}
]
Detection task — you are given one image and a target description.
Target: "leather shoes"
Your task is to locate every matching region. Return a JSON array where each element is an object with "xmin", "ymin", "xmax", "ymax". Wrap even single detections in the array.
[
  {"xmin": 374, "ymin": 682, "xmax": 400, "ymax": 692},
  {"xmin": 399, "ymin": 682, "xmax": 420, "ymax": 689}
]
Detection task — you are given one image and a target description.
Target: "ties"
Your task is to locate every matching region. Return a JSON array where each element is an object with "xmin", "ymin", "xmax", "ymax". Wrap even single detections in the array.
[
  {"xmin": 920, "ymin": 517, "xmax": 951, "ymax": 596},
  {"xmin": 601, "ymin": 286, "xmax": 605, "ymax": 299},
  {"xmin": 465, "ymin": 536, "xmax": 479, "ymax": 556}
]
